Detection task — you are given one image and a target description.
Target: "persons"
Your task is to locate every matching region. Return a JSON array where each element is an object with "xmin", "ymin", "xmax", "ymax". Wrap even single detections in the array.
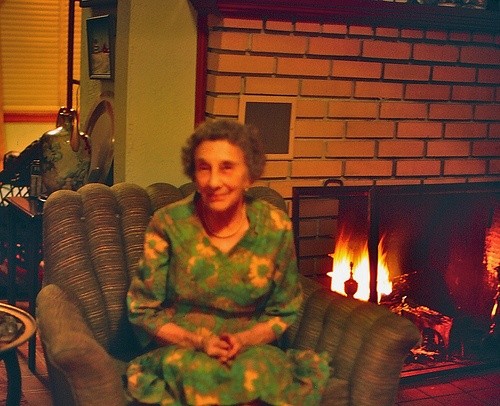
[{"xmin": 127, "ymin": 120, "xmax": 333, "ymax": 406}]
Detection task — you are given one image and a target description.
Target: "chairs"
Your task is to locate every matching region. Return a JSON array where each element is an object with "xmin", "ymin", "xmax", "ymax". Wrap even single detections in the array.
[{"xmin": 0, "ymin": 123, "xmax": 93, "ymax": 306}]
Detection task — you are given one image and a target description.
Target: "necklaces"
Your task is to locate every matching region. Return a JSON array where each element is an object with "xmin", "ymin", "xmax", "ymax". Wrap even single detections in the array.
[{"xmin": 202, "ymin": 196, "xmax": 246, "ymax": 238}]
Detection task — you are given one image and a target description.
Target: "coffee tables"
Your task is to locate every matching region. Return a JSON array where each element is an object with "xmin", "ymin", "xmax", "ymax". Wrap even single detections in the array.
[{"xmin": 0, "ymin": 303, "xmax": 37, "ymax": 406}]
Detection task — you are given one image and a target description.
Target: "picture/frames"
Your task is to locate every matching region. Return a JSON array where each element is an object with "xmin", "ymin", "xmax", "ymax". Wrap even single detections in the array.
[{"xmin": 85, "ymin": 15, "xmax": 116, "ymax": 82}]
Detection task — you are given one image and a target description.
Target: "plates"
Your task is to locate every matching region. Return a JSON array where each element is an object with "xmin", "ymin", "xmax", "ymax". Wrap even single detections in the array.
[{"xmin": 84, "ymin": 90, "xmax": 115, "ymax": 185}]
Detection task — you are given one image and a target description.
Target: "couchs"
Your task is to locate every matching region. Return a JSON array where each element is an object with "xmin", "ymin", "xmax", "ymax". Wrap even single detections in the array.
[{"xmin": 35, "ymin": 182, "xmax": 420, "ymax": 406}]
